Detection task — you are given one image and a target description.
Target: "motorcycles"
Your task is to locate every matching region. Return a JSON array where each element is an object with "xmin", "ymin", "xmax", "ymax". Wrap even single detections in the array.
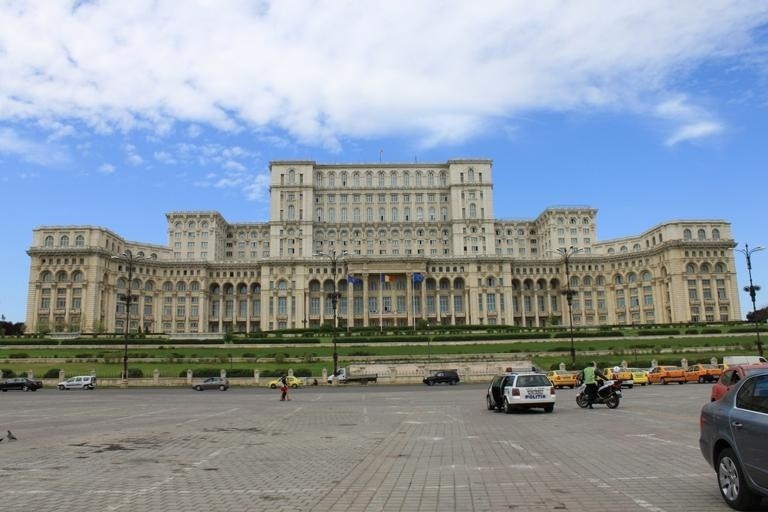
[{"xmin": 575, "ymin": 378, "xmax": 623, "ymax": 409}]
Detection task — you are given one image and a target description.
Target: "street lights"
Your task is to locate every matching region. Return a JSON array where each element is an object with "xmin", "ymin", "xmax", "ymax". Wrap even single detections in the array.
[
  {"xmin": 727, "ymin": 242, "xmax": 765, "ymax": 355},
  {"xmin": 110, "ymin": 249, "xmax": 158, "ymax": 383},
  {"xmin": 546, "ymin": 244, "xmax": 588, "ymax": 370},
  {"xmin": 311, "ymin": 249, "xmax": 354, "ymax": 376}
]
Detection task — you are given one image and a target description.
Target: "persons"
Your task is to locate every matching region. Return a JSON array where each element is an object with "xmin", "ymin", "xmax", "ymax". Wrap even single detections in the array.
[
  {"xmin": 279, "ymin": 384, "xmax": 288, "ymax": 401},
  {"xmin": 282, "ymin": 376, "xmax": 287, "ymax": 385},
  {"xmin": 578, "ymin": 361, "xmax": 607, "ymax": 408},
  {"xmin": 312, "ymin": 378, "xmax": 318, "ymax": 385}
]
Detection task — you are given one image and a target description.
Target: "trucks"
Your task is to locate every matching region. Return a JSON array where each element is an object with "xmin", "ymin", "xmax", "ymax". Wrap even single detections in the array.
[
  {"xmin": 720, "ymin": 356, "xmax": 767, "ymax": 369},
  {"xmin": 327, "ymin": 367, "xmax": 377, "ymax": 385}
]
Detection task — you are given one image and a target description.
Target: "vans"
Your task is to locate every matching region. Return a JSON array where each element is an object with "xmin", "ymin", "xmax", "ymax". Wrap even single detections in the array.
[{"xmin": 55, "ymin": 375, "xmax": 96, "ymax": 391}]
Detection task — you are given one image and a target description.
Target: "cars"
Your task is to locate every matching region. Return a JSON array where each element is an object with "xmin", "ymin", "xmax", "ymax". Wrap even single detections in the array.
[
  {"xmin": 710, "ymin": 364, "xmax": 767, "ymax": 404},
  {"xmin": 485, "ymin": 366, "xmax": 556, "ymax": 413},
  {"xmin": 626, "ymin": 368, "xmax": 648, "ymax": 386},
  {"xmin": 544, "ymin": 370, "xmax": 574, "ymax": 389},
  {"xmin": 697, "ymin": 368, "xmax": 767, "ymax": 511},
  {"xmin": 714, "ymin": 364, "xmax": 736, "ymax": 374},
  {"xmin": 647, "ymin": 365, "xmax": 685, "ymax": 385},
  {"xmin": 268, "ymin": 375, "xmax": 304, "ymax": 389},
  {"xmin": 682, "ymin": 364, "xmax": 722, "ymax": 383},
  {"xmin": 602, "ymin": 367, "xmax": 633, "ymax": 388},
  {"xmin": 0, "ymin": 377, "xmax": 43, "ymax": 393}
]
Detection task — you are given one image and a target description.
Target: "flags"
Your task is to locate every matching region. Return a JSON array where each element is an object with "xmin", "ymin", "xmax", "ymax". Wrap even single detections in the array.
[
  {"xmin": 348, "ymin": 275, "xmax": 359, "ymax": 287},
  {"xmin": 413, "ymin": 272, "xmax": 424, "ymax": 283},
  {"xmin": 380, "ymin": 273, "xmax": 394, "ymax": 283}
]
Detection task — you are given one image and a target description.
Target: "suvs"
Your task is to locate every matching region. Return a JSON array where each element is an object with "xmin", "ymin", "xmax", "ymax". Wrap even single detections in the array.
[
  {"xmin": 422, "ymin": 370, "xmax": 459, "ymax": 386},
  {"xmin": 192, "ymin": 377, "xmax": 229, "ymax": 392}
]
